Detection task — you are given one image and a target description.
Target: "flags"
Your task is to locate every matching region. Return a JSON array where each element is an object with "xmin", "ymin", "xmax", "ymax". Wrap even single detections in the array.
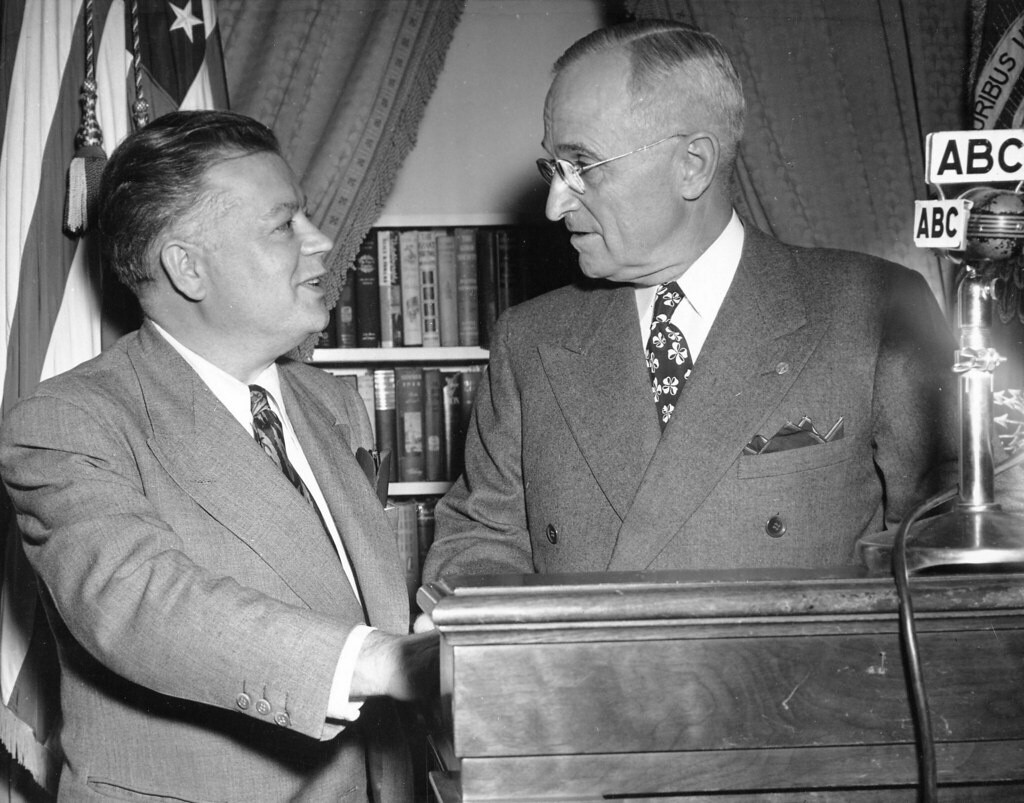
[{"xmin": 0, "ymin": 0, "xmax": 229, "ymax": 803}]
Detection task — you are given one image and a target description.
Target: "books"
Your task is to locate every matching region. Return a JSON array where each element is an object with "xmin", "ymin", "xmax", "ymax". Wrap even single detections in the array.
[{"xmin": 303, "ymin": 228, "xmax": 577, "ymax": 628}]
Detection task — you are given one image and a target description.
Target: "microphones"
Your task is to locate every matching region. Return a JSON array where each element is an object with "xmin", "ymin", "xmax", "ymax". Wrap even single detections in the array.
[{"xmin": 949, "ymin": 185, "xmax": 1024, "ymax": 331}]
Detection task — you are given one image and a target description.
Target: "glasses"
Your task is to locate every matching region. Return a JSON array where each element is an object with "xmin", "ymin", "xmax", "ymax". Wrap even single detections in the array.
[{"xmin": 537, "ymin": 135, "xmax": 688, "ymax": 195}]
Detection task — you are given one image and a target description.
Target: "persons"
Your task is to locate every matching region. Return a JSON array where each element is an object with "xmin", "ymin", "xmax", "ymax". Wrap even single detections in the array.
[
  {"xmin": 1, "ymin": 110, "xmax": 439, "ymax": 803},
  {"xmin": 414, "ymin": 21, "xmax": 1024, "ymax": 635}
]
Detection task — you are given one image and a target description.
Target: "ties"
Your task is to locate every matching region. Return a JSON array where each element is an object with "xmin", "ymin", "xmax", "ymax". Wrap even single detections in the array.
[
  {"xmin": 249, "ymin": 385, "xmax": 343, "ymax": 566},
  {"xmin": 645, "ymin": 281, "xmax": 693, "ymax": 434}
]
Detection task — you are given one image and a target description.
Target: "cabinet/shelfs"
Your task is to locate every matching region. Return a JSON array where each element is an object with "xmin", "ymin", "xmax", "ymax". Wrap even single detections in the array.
[{"xmin": 304, "ymin": 214, "xmax": 565, "ymax": 495}]
